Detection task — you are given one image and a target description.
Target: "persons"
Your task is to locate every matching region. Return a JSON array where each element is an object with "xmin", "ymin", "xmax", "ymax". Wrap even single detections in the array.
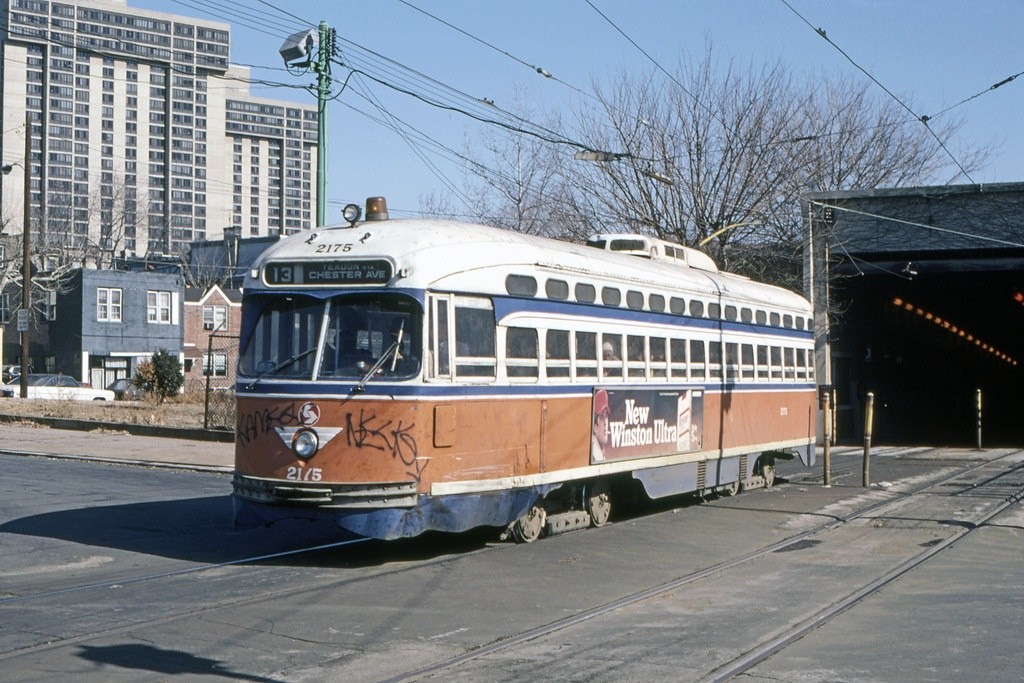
[
  {"xmin": 603, "ymin": 342, "xmax": 621, "ymax": 376},
  {"xmin": 592, "ymin": 389, "xmax": 610, "ymax": 461},
  {"xmin": 416, "ymin": 320, "xmax": 474, "ymax": 376}
]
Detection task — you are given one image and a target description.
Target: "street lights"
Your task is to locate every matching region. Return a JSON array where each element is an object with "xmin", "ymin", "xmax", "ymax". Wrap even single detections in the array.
[{"xmin": 0, "ymin": 163, "xmax": 31, "ymax": 399}]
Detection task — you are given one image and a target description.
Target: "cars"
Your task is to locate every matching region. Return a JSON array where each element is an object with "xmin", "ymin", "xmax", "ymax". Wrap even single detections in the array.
[
  {"xmin": 6, "ymin": 373, "xmax": 80, "ymax": 387},
  {"xmin": 106, "ymin": 378, "xmax": 147, "ymax": 401},
  {"xmin": 0, "ymin": 365, "xmax": 32, "ymax": 398}
]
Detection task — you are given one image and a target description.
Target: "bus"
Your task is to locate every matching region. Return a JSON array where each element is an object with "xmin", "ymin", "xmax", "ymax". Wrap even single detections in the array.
[{"xmin": 232, "ymin": 197, "xmax": 816, "ymax": 545}]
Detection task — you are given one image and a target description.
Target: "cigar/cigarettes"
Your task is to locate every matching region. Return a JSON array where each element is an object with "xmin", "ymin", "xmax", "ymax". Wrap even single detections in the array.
[{"xmin": 604, "ymin": 431, "xmax": 610, "ymax": 434}]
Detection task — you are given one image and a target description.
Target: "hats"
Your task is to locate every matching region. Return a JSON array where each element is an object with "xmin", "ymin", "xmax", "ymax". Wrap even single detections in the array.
[
  {"xmin": 603, "ymin": 343, "xmax": 613, "ymax": 352},
  {"xmin": 595, "ymin": 389, "xmax": 610, "ymax": 415}
]
